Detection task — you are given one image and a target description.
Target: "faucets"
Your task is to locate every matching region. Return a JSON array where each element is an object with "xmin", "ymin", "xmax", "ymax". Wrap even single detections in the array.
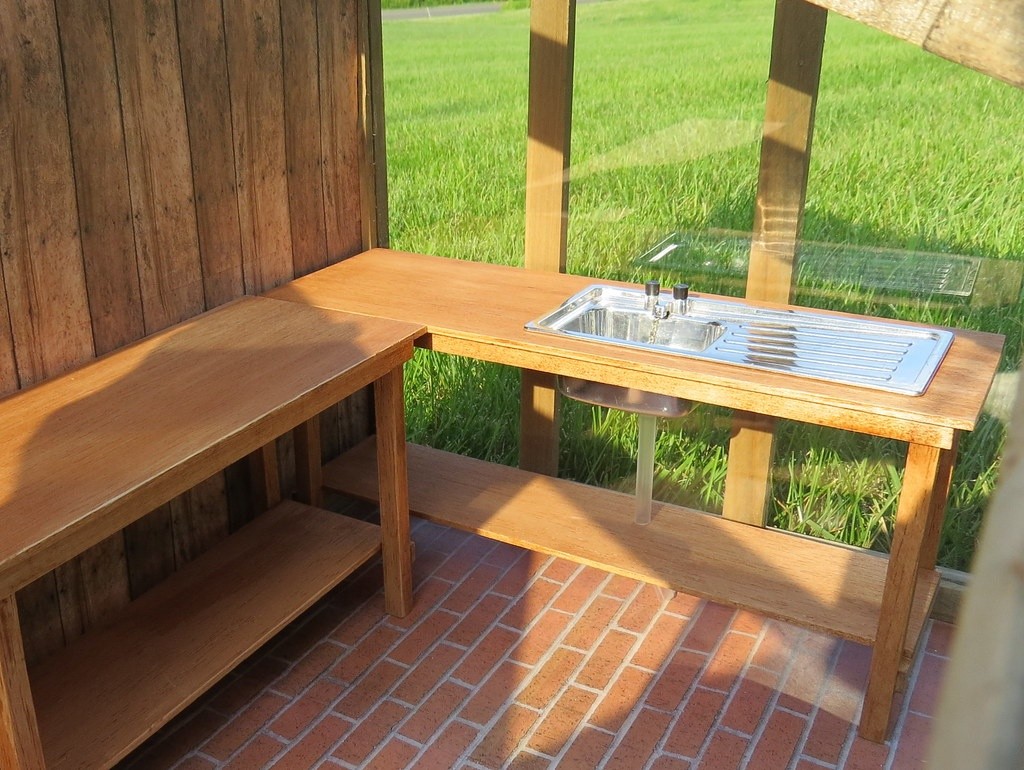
[{"xmin": 643, "ymin": 281, "xmax": 689, "ymax": 319}]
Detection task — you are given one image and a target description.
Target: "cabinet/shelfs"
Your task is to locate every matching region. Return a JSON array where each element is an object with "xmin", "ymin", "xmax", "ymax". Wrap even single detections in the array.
[{"xmin": 0, "ymin": 294, "xmax": 427, "ymax": 769}]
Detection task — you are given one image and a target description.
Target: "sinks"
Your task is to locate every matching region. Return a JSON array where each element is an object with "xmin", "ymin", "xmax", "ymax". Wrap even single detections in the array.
[{"xmin": 523, "ymin": 283, "xmax": 726, "ymax": 419}]
